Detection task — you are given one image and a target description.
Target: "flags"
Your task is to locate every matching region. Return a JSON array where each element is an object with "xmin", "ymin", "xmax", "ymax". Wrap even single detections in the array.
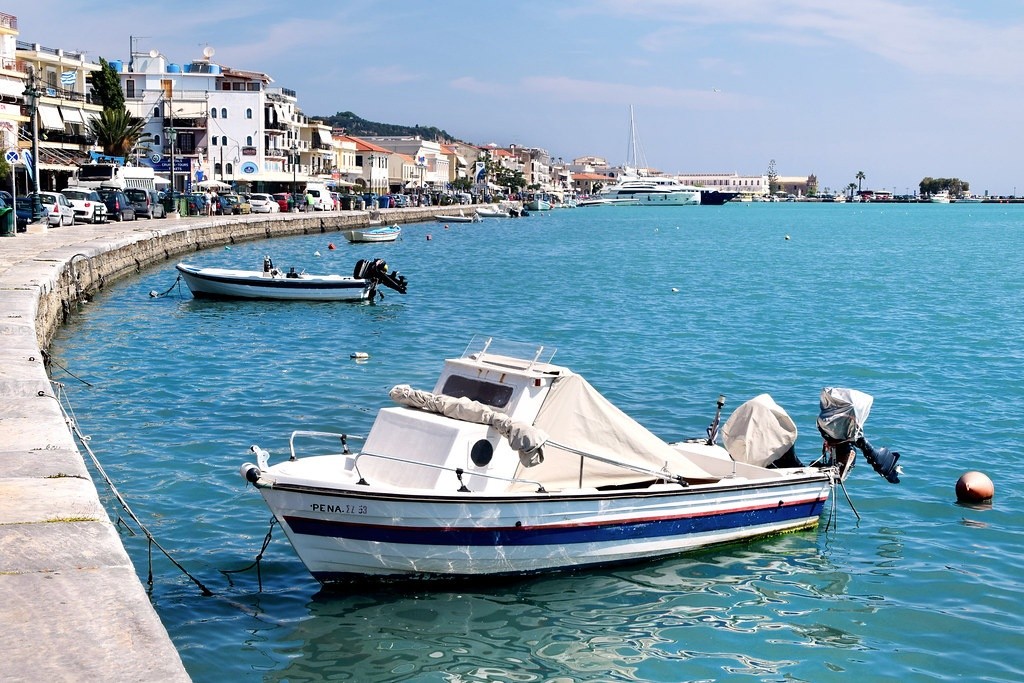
[{"xmin": 61, "ymin": 71, "xmax": 77, "ymax": 85}]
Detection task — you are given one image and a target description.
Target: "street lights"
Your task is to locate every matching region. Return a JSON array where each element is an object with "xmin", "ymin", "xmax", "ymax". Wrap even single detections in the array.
[
  {"xmin": 21, "ymin": 65, "xmax": 40, "ymax": 194},
  {"xmin": 368, "ymin": 155, "xmax": 375, "ymax": 196},
  {"xmin": 290, "ymin": 144, "xmax": 300, "ymax": 213},
  {"xmin": 416, "ymin": 163, "xmax": 428, "ymax": 190},
  {"xmin": 168, "ymin": 97, "xmax": 184, "ymax": 209}
]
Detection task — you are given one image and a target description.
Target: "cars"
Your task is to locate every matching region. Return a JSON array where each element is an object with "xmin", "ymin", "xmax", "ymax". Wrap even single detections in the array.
[
  {"xmin": 224, "ymin": 194, "xmax": 253, "ymax": 215},
  {"xmin": 2, "ymin": 198, "xmax": 49, "ymax": 226},
  {"xmin": 273, "ymin": 188, "xmax": 521, "ymax": 210},
  {"xmin": 26, "ymin": 192, "xmax": 76, "ymax": 228},
  {"xmin": 173, "ymin": 195, "xmax": 207, "ymax": 217},
  {"xmin": 0, "ymin": 190, "xmax": 14, "ymax": 214},
  {"xmin": 61, "ymin": 188, "xmax": 109, "ymax": 224},
  {"xmin": 212, "ymin": 195, "xmax": 234, "ymax": 216},
  {"xmin": 124, "ymin": 187, "xmax": 166, "ymax": 220},
  {"xmin": 248, "ymin": 192, "xmax": 281, "ymax": 213},
  {"xmin": 92, "ymin": 185, "xmax": 138, "ymax": 221}
]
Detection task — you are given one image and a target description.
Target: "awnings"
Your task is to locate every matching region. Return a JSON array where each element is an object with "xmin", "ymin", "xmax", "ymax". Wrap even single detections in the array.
[
  {"xmin": 38, "ymin": 104, "xmax": 88, "ymax": 130},
  {"xmin": 0, "ymin": 75, "xmax": 26, "ymax": 99}
]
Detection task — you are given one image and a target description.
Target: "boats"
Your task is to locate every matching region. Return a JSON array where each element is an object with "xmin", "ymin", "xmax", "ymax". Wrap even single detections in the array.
[
  {"xmin": 576, "ymin": 194, "xmax": 642, "ymax": 208},
  {"xmin": 176, "ymin": 255, "xmax": 410, "ymax": 303},
  {"xmin": 729, "ymin": 190, "xmax": 988, "ymax": 202},
  {"xmin": 238, "ymin": 334, "xmax": 902, "ymax": 587},
  {"xmin": 600, "ymin": 180, "xmax": 702, "ymax": 207},
  {"xmin": 527, "ymin": 192, "xmax": 554, "ymax": 211},
  {"xmin": 341, "ymin": 224, "xmax": 402, "ymax": 245},
  {"xmin": 475, "ymin": 205, "xmax": 530, "ymax": 219},
  {"xmin": 434, "ymin": 209, "xmax": 484, "ymax": 224},
  {"xmin": 559, "ymin": 191, "xmax": 578, "ymax": 209}
]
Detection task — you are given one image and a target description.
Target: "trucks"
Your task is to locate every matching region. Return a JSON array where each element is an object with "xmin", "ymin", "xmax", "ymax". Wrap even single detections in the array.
[{"xmin": 73, "ymin": 163, "xmax": 155, "ymax": 192}]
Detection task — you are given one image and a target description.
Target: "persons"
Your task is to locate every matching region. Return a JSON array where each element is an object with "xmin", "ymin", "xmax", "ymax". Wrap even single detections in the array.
[{"xmin": 205, "ymin": 189, "xmax": 212, "ymax": 217}]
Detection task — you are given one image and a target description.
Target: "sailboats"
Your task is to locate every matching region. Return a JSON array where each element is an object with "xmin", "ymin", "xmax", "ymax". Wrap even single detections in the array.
[{"xmin": 597, "ymin": 104, "xmax": 743, "ymax": 206}]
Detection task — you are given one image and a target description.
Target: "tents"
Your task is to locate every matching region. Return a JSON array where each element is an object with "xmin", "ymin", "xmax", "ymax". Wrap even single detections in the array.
[
  {"xmin": 154, "ymin": 175, "xmax": 171, "ymax": 190},
  {"xmin": 194, "ymin": 180, "xmax": 232, "ymax": 191}
]
